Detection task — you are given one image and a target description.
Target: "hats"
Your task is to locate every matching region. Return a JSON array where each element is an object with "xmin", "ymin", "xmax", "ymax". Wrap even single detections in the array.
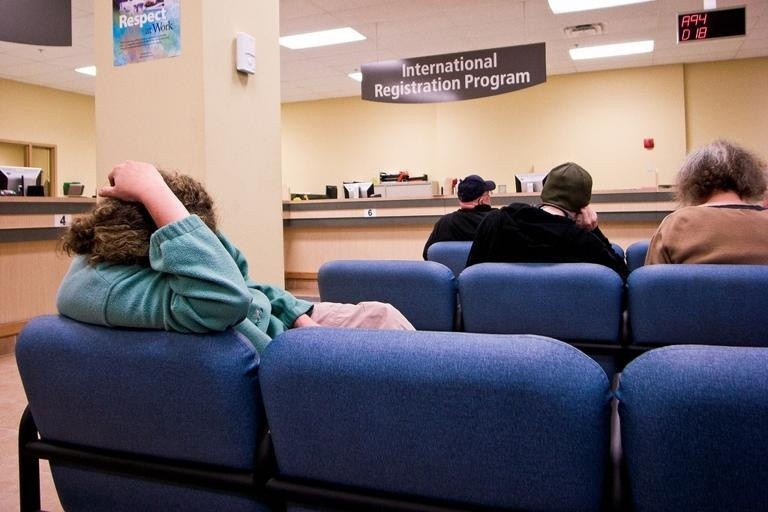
[
  {"xmin": 540, "ymin": 162, "xmax": 592, "ymax": 213},
  {"xmin": 458, "ymin": 175, "xmax": 496, "ymax": 202}
]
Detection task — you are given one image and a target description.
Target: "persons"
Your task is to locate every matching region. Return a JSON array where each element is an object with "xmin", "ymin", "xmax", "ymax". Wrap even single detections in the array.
[
  {"xmin": 643, "ymin": 137, "xmax": 767, "ymax": 264},
  {"xmin": 421, "ymin": 174, "xmax": 497, "ymax": 262},
  {"xmin": 53, "ymin": 159, "xmax": 419, "ymax": 358},
  {"xmin": 462, "ymin": 162, "xmax": 632, "ymax": 286}
]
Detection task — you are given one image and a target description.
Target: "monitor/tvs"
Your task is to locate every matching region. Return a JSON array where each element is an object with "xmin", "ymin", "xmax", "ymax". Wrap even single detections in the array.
[
  {"xmin": 515, "ymin": 173, "xmax": 548, "ymax": 193},
  {"xmin": 343, "ymin": 182, "xmax": 374, "ymax": 198},
  {"xmin": 0, "ymin": 166, "xmax": 43, "ymax": 195},
  {"xmin": 326, "ymin": 186, "xmax": 337, "ymax": 199}
]
